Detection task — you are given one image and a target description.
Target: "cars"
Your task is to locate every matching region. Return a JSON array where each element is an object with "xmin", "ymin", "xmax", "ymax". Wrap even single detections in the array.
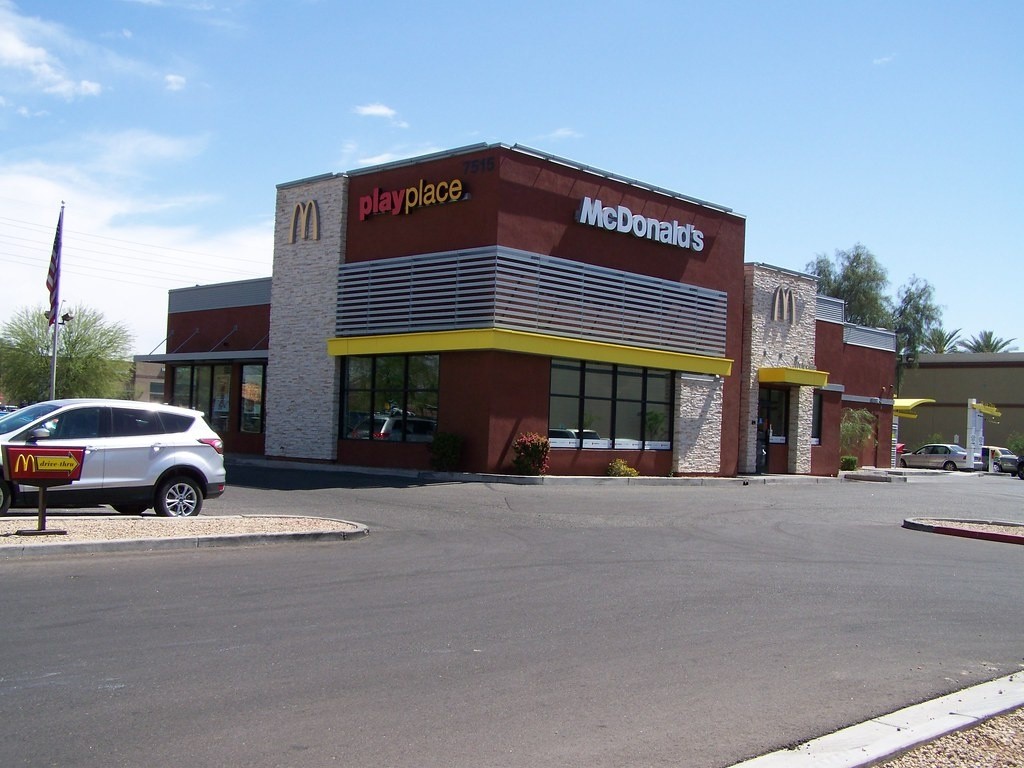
[
  {"xmin": 1016, "ymin": 456, "xmax": 1024, "ymax": 480},
  {"xmin": 982, "ymin": 446, "xmax": 1019, "ymax": 476},
  {"xmin": 0, "ymin": 404, "xmax": 26, "ymax": 417},
  {"xmin": 901, "ymin": 443, "xmax": 983, "ymax": 471}
]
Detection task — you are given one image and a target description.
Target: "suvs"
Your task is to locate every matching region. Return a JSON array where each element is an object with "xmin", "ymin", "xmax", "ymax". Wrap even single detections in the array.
[
  {"xmin": 548, "ymin": 428, "xmax": 599, "ymax": 439},
  {"xmin": 348, "ymin": 413, "xmax": 436, "ymax": 442},
  {"xmin": 0, "ymin": 397, "xmax": 226, "ymax": 517}
]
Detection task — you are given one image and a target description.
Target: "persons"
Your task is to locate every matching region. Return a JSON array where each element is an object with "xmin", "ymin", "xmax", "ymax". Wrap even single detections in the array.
[
  {"xmin": 19, "ymin": 400, "xmax": 28, "ymax": 409},
  {"xmin": 0, "ymin": 402, "xmax": 6, "ymax": 412}
]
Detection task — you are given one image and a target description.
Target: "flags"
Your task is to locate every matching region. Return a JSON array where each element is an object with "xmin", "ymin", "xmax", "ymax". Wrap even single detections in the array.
[{"xmin": 46, "ymin": 212, "xmax": 61, "ymax": 327}]
